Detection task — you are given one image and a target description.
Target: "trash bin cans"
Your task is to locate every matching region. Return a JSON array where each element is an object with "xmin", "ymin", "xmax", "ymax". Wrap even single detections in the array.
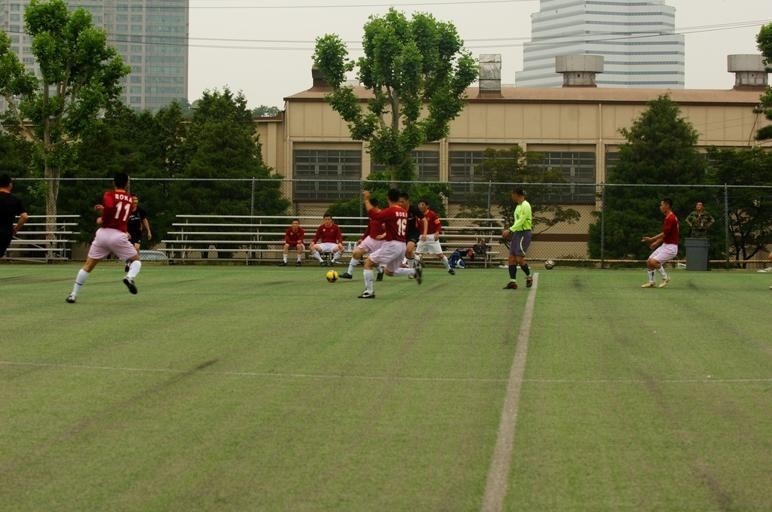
[{"xmin": 684, "ymin": 238, "xmax": 712, "ymax": 271}]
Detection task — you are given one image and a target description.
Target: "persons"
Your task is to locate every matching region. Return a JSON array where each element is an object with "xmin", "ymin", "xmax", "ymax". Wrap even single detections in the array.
[
  {"xmin": 123, "ymin": 193, "xmax": 153, "ymax": 273},
  {"xmin": 276, "ymin": 218, "xmax": 306, "ymax": 267},
  {"xmin": 338, "ymin": 187, "xmax": 455, "ymax": 298},
  {"xmin": 66, "ymin": 171, "xmax": 142, "ymax": 303},
  {"xmin": 684, "ymin": 200, "xmax": 716, "ymax": 231},
  {"xmin": 308, "ymin": 214, "xmax": 346, "ymax": 267},
  {"xmin": 500, "ymin": 187, "xmax": 534, "ymax": 290},
  {"xmin": 0, "ymin": 173, "xmax": 29, "ymax": 257},
  {"xmin": 639, "ymin": 197, "xmax": 681, "ymax": 289}
]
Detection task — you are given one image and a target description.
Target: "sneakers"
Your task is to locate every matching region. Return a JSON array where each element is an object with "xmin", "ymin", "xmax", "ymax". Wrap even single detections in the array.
[
  {"xmin": 641, "ymin": 278, "xmax": 670, "ymax": 288},
  {"xmin": 503, "ymin": 282, "xmax": 517, "ymax": 288},
  {"xmin": 527, "ymin": 277, "xmax": 533, "ymax": 286},
  {"xmin": 339, "ymin": 272, "xmax": 352, "ymax": 278},
  {"xmin": 123, "ymin": 277, "xmax": 136, "ymax": 294}
]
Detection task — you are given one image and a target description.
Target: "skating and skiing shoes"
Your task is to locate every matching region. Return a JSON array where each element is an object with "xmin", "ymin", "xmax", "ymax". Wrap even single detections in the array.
[{"xmin": 377, "ymin": 264, "xmax": 421, "ymax": 284}]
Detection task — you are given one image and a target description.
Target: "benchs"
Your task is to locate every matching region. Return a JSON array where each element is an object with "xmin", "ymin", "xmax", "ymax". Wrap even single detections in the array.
[
  {"xmin": 0, "ymin": 215, "xmax": 81, "ymax": 264},
  {"xmin": 157, "ymin": 215, "xmax": 505, "ymax": 268}
]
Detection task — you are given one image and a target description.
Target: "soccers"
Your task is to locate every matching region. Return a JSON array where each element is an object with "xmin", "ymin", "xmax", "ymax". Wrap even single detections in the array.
[
  {"xmin": 545, "ymin": 261, "xmax": 554, "ymax": 269},
  {"xmin": 326, "ymin": 270, "xmax": 339, "ymax": 282}
]
